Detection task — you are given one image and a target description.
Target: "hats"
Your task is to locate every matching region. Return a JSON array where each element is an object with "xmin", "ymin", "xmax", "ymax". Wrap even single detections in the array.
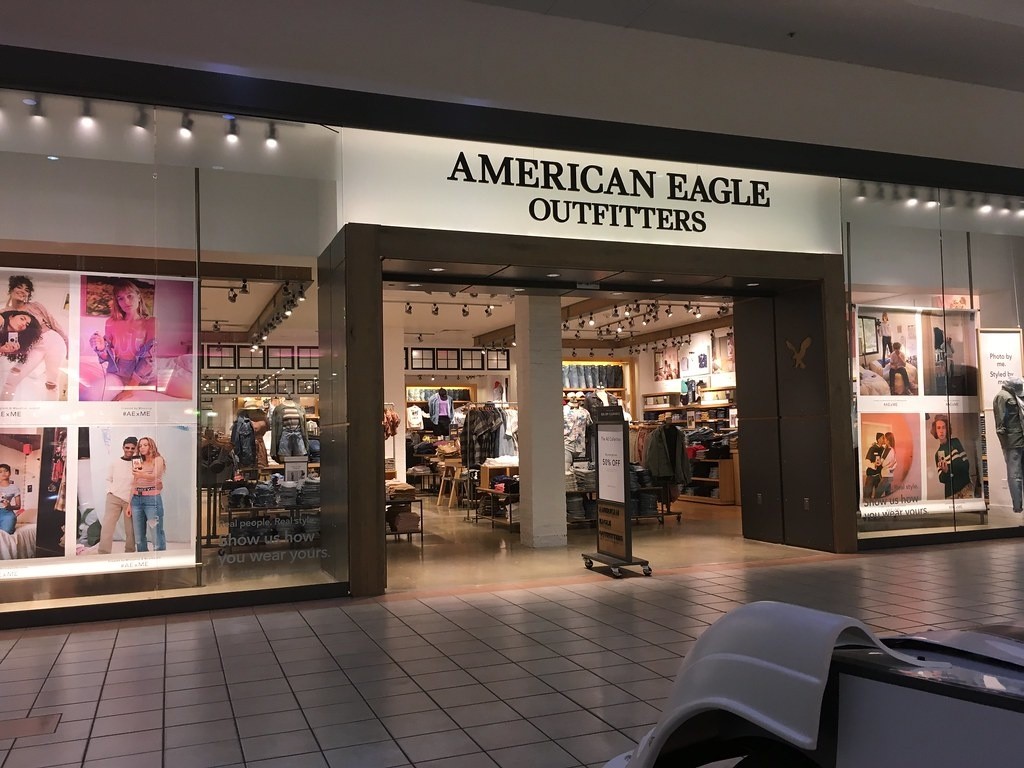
[{"xmin": 1008, "ymin": 376, "xmax": 1024, "ymax": 396}]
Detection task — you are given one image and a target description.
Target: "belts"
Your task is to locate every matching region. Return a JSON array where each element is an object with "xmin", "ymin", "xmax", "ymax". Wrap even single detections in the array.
[{"xmin": 133, "ymin": 489, "xmax": 159, "ymax": 496}]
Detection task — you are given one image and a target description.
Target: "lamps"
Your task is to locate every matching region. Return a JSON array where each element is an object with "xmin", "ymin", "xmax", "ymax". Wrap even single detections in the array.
[
  {"xmin": 133, "ymin": 110, "xmax": 151, "ymax": 128},
  {"xmin": 927, "ymin": 194, "xmax": 938, "ymax": 208},
  {"xmin": 222, "ymin": 117, "xmax": 239, "ymax": 142},
  {"xmin": 857, "ymin": 186, "xmax": 866, "ymax": 200},
  {"xmin": 943, "ymin": 196, "xmax": 955, "ymax": 209},
  {"xmin": 892, "ymin": 189, "xmax": 903, "ymax": 200},
  {"xmin": 211, "ymin": 279, "xmax": 734, "ymax": 381},
  {"xmin": 1017, "ymin": 204, "xmax": 1024, "ymax": 215},
  {"xmin": 876, "ymin": 188, "xmax": 884, "ymax": 200},
  {"xmin": 266, "ymin": 123, "xmax": 279, "ymax": 147},
  {"xmin": 29, "ymin": 93, "xmax": 47, "ymax": 119},
  {"xmin": 180, "ymin": 113, "xmax": 195, "ymax": 137},
  {"xmin": 966, "ymin": 196, "xmax": 976, "ymax": 208},
  {"xmin": 980, "ymin": 197, "xmax": 991, "ymax": 211},
  {"xmin": 907, "ymin": 191, "xmax": 918, "ymax": 207},
  {"xmin": 79, "ymin": 101, "xmax": 93, "ymax": 120},
  {"xmin": 1001, "ymin": 202, "xmax": 1011, "ymax": 212}
]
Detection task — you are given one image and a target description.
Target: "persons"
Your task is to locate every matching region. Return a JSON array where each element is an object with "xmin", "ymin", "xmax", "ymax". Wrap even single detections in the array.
[
  {"xmin": 229, "ymin": 399, "xmax": 270, "ymax": 480},
  {"xmin": 0, "ymin": 275, "xmax": 69, "ymax": 401},
  {"xmin": 582, "ymin": 382, "xmax": 618, "ymax": 463},
  {"xmin": 428, "ymin": 388, "xmax": 454, "ymax": 438},
  {"xmin": 726, "ymin": 335, "xmax": 736, "ymax": 372},
  {"xmin": 874, "ymin": 432, "xmax": 896, "ymax": 500},
  {"xmin": 889, "ymin": 341, "xmax": 915, "ymax": 396},
  {"xmin": 125, "ymin": 437, "xmax": 167, "ymax": 553},
  {"xmin": 0, "ymin": 305, "xmax": 43, "ymax": 363},
  {"xmin": 929, "ymin": 414, "xmax": 976, "ymax": 500},
  {"xmin": 862, "ymin": 432, "xmax": 886, "ymax": 502},
  {"xmin": 89, "ymin": 280, "xmax": 193, "ymax": 402},
  {"xmin": 940, "ymin": 336, "xmax": 958, "ymax": 390},
  {"xmin": 270, "ymin": 392, "xmax": 309, "ymax": 464},
  {"xmin": 876, "ymin": 311, "xmax": 894, "ymax": 369},
  {"xmin": 0, "ymin": 463, "xmax": 21, "ymax": 536},
  {"xmin": 653, "ymin": 359, "xmax": 681, "ymax": 382},
  {"xmin": 97, "ymin": 435, "xmax": 143, "ymax": 555},
  {"xmin": 993, "ymin": 378, "xmax": 1024, "ymax": 513},
  {"xmin": 562, "ymin": 396, "xmax": 594, "ymax": 473}
]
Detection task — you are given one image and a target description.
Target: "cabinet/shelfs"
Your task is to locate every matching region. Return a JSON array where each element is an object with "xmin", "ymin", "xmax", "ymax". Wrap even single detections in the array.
[{"xmin": 203, "ymin": 385, "xmax": 740, "ymax": 553}]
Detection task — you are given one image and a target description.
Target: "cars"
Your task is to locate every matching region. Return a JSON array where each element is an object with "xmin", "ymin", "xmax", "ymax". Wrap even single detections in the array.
[{"xmin": 602, "ymin": 597, "xmax": 1023, "ymax": 768}]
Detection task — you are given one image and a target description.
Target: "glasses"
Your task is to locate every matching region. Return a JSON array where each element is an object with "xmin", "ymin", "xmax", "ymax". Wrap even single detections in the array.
[{"xmin": 124, "ymin": 446, "xmax": 136, "ymax": 450}]
